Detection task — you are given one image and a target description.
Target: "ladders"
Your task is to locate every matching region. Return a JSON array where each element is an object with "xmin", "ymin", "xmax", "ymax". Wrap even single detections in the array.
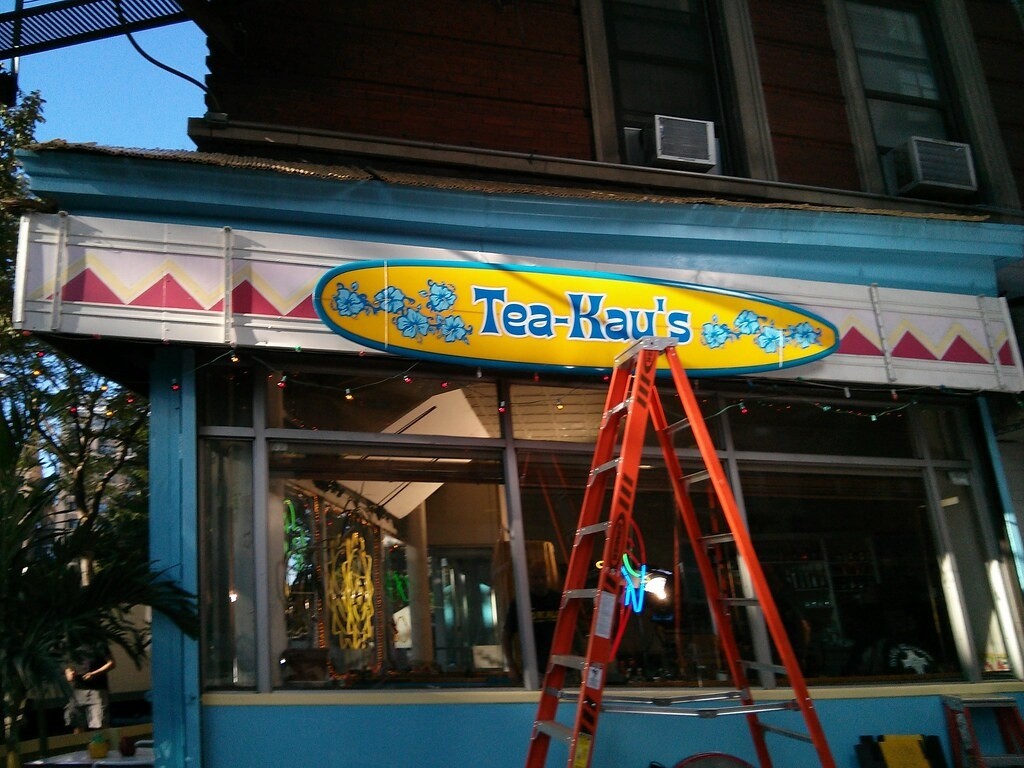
[
  {"xmin": 944, "ymin": 693, "xmax": 1024, "ymax": 768},
  {"xmin": 525, "ymin": 335, "xmax": 834, "ymax": 768}
]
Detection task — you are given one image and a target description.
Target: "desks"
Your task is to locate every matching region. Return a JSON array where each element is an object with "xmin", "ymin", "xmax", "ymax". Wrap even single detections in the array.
[{"xmin": 25, "ymin": 747, "xmax": 155, "ymax": 765}]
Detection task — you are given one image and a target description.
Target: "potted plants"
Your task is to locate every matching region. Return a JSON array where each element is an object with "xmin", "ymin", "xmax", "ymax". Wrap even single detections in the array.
[{"xmin": 88, "ymin": 731, "xmax": 109, "ymax": 758}]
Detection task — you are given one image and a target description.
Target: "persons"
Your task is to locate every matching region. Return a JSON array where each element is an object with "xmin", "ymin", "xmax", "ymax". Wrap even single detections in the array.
[
  {"xmin": 502, "ymin": 561, "xmax": 586, "ymax": 687},
  {"xmin": 64, "ymin": 647, "xmax": 116, "ymax": 732},
  {"xmin": 770, "ymin": 576, "xmax": 811, "ymax": 677}
]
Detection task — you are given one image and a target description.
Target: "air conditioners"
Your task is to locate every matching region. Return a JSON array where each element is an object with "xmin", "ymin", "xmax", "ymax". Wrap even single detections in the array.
[
  {"xmin": 643, "ymin": 114, "xmax": 717, "ymax": 173},
  {"xmin": 886, "ymin": 135, "xmax": 978, "ymax": 196}
]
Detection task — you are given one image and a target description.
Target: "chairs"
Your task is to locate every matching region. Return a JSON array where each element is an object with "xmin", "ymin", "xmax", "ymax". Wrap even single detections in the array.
[
  {"xmin": 134, "ymin": 739, "xmax": 154, "ymax": 748},
  {"xmin": 92, "ymin": 761, "xmax": 155, "ymax": 768}
]
